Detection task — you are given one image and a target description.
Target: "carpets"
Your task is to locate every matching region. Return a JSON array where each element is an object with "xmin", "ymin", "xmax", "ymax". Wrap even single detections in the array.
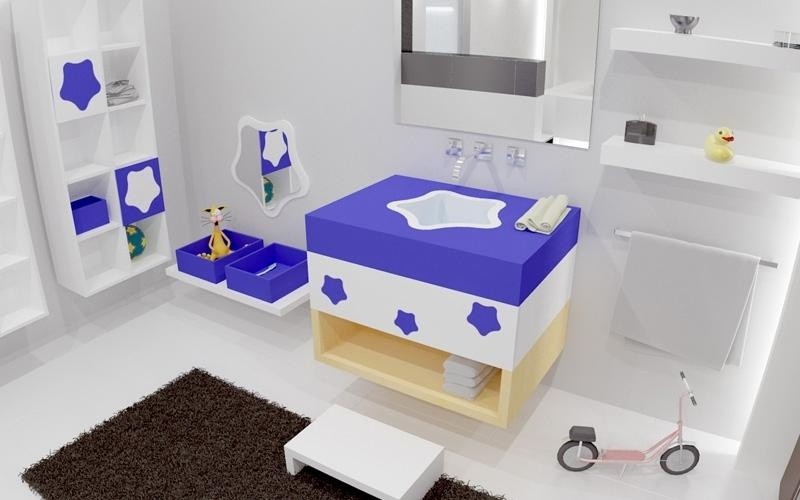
[{"xmin": 17, "ymin": 367, "xmax": 508, "ymax": 498}]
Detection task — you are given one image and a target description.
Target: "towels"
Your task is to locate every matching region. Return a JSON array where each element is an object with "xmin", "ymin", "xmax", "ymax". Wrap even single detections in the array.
[
  {"xmin": 609, "ymin": 230, "xmax": 762, "ymax": 373},
  {"xmin": 441, "ymin": 356, "xmax": 502, "ymax": 402}
]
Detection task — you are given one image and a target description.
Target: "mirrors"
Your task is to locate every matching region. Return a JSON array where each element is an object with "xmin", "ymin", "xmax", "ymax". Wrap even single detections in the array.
[
  {"xmin": 394, "ymin": 0, "xmax": 602, "ymax": 150},
  {"xmin": 230, "ymin": 115, "xmax": 311, "ymax": 221}
]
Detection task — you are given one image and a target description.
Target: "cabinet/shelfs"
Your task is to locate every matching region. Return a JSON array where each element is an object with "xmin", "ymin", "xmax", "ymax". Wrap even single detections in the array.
[
  {"xmin": 0, "ymin": 65, "xmax": 52, "ymax": 337},
  {"xmin": 9, "ymin": 0, "xmax": 173, "ymax": 298}
]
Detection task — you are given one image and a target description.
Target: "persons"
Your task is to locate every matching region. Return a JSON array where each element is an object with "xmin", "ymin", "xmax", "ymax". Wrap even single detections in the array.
[{"xmin": 703, "ymin": 126, "xmax": 735, "ymax": 164}]
